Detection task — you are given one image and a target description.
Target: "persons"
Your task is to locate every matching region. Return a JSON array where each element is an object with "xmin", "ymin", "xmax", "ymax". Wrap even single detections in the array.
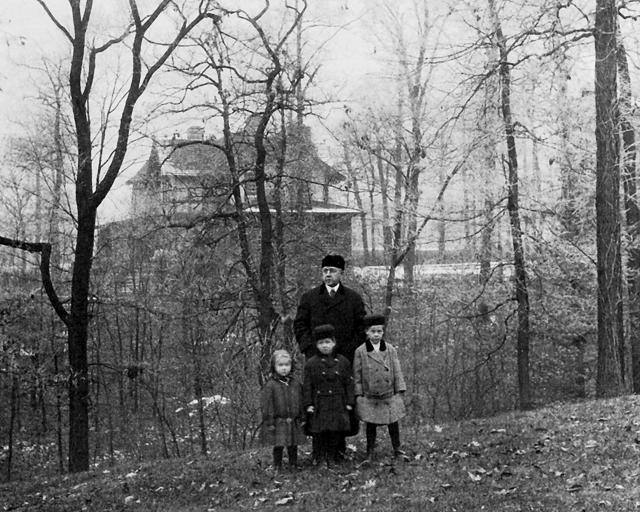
[
  {"xmin": 301, "ymin": 324, "xmax": 356, "ymax": 468},
  {"xmin": 263, "ymin": 349, "xmax": 308, "ymax": 477},
  {"xmin": 292, "ymin": 255, "xmax": 368, "ymax": 464},
  {"xmin": 353, "ymin": 313, "xmax": 408, "ymax": 466}
]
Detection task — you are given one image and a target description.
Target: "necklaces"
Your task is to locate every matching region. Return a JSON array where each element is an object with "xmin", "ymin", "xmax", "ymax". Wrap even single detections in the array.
[{"xmin": 278, "ymin": 377, "xmax": 290, "ymax": 387}]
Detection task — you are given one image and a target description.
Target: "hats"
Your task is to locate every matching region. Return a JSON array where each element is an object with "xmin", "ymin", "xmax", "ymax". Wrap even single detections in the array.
[
  {"xmin": 364, "ymin": 315, "xmax": 385, "ymax": 327},
  {"xmin": 322, "ymin": 255, "xmax": 344, "ymax": 270},
  {"xmin": 313, "ymin": 325, "xmax": 335, "ymax": 340}
]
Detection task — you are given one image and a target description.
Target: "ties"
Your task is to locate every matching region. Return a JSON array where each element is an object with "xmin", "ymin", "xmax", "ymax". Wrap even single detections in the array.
[{"xmin": 330, "ymin": 290, "xmax": 336, "ymax": 300}]
